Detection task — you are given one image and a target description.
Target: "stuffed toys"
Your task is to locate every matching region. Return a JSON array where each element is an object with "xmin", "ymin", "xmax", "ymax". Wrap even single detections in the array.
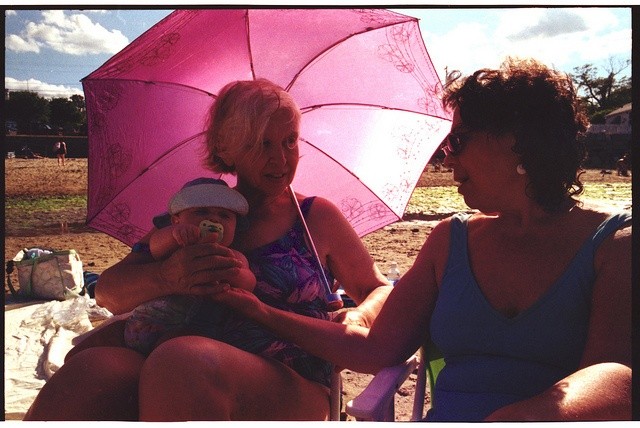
[{"xmin": 197, "ymin": 218, "xmax": 224, "ymax": 244}]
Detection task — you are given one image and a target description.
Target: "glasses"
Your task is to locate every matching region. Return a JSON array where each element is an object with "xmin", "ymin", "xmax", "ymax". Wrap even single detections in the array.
[{"xmin": 440, "ymin": 129, "xmax": 476, "ymax": 157}]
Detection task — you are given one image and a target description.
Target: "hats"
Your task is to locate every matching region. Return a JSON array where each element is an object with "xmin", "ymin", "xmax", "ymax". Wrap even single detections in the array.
[{"xmin": 169, "ymin": 178, "xmax": 249, "ymax": 216}]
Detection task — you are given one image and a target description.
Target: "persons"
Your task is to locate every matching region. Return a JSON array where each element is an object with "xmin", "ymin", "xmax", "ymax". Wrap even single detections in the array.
[
  {"xmin": 209, "ymin": 54, "xmax": 631, "ymax": 421},
  {"xmin": 124, "ymin": 177, "xmax": 258, "ymax": 353},
  {"xmin": 21, "ymin": 79, "xmax": 395, "ymax": 421},
  {"xmin": 434, "ymin": 145, "xmax": 451, "ymax": 172},
  {"xmin": 617, "ymin": 155, "xmax": 629, "ymax": 177},
  {"xmin": 56, "ymin": 139, "xmax": 67, "ymax": 167}
]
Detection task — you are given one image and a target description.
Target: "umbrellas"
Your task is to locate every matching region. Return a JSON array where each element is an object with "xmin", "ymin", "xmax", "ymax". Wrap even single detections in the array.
[{"xmin": 79, "ymin": 9, "xmax": 455, "ymax": 312}]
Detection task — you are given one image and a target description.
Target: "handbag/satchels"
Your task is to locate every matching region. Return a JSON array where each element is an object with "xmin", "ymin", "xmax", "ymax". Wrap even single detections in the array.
[{"xmin": 6, "ymin": 248, "xmax": 84, "ymax": 300}]
